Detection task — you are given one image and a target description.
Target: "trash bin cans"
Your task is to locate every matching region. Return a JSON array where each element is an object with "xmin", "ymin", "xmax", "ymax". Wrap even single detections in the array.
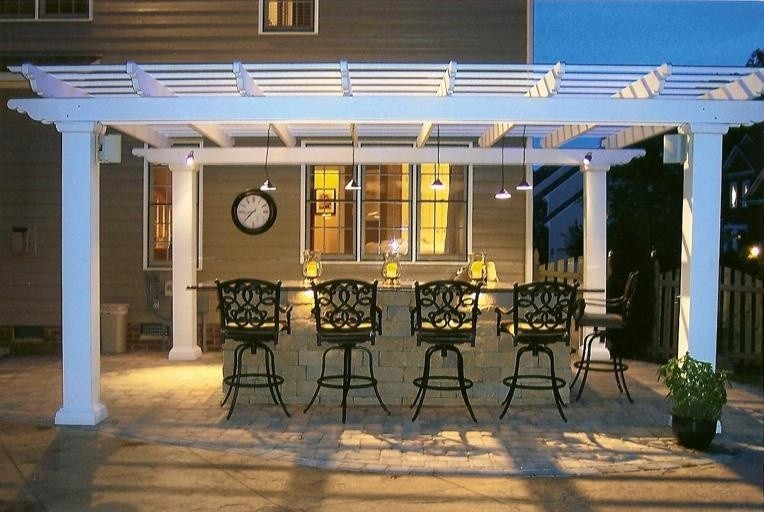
[{"xmin": 101, "ymin": 302, "xmax": 130, "ymax": 354}]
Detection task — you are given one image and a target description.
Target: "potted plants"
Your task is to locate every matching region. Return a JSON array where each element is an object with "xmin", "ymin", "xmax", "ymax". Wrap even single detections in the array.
[{"xmin": 655, "ymin": 350, "xmax": 734, "ymax": 451}]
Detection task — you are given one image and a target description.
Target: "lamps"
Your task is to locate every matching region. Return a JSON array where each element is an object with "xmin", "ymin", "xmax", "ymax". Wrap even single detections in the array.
[{"xmin": 259, "ymin": 123, "xmax": 532, "ymax": 199}]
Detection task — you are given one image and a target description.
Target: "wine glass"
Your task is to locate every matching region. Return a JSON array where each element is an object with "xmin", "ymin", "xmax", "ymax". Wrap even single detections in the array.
[
  {"xmin": 379, "ymin": 251, "xmax": 404, "ymax": 289},
  {"xmin": 300, "ymin": 249, "xmax": 322, "ymax": 288},
  {"xmin": 466, "ymin": 253, "xmax": 487, "ymax": 285}
]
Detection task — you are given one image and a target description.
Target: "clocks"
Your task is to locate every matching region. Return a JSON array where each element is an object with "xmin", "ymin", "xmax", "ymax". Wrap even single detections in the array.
[{"xmin": 229, "ymin": 188, "xmax": 278, "ymax": 237}]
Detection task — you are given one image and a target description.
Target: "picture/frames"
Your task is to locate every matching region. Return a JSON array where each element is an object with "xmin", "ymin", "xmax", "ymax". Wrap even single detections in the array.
[{"xmin": 314, "ymin": 187, "xmax": 336, "ymax": 216}]
